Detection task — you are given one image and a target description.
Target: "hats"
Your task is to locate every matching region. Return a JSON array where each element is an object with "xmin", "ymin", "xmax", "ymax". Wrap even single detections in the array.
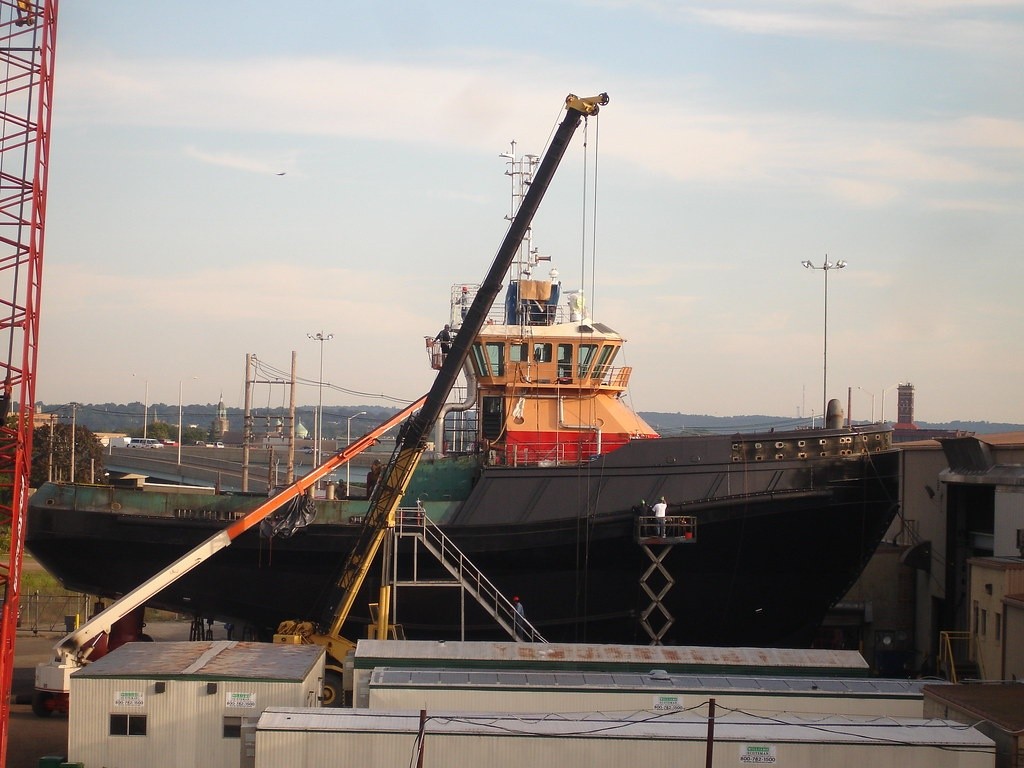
[
  {"xmin": 513, "ymin": 596, "xmax": 519, "ymax": 601},
  {"xmin": 659, "ymin": 497, "xmax": 664, "ymax": 500}
]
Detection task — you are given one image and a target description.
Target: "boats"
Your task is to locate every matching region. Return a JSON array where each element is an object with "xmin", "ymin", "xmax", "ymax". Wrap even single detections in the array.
[{"xmin": 20, "ymin": 142, "xmax": 900, "ymax": 650}]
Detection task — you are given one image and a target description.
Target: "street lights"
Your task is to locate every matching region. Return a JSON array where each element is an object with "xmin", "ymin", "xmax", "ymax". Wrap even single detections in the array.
[
  {"xmin": 803, "ymin": 253, "xmax": 848, "ymax": 428},
  {"xmin": 177, "ymin": 375, "xmax": 198, "ymax": 465},
  {"xmin": 307, "ymin": 330, "xmax": 335, "ymax": 490},
  {"xmin": 347, "ymin": 411, "xmax": 367, "ymax": 496}
]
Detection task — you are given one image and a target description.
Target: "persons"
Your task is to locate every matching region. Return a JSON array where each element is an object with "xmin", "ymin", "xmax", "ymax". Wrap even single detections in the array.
[
  {"xmin": 456, "ymin": 287, "xmax": 471, "ymax": 320},
  {"xmin": 433, "ymin": 324, "xmax": 451, "ymax": 364}
]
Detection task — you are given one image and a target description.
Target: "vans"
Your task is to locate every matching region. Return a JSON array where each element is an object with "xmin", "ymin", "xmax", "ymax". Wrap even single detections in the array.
[{"xmin": 128, "ymin": 437, "xmax": 165, "ymax": 449}]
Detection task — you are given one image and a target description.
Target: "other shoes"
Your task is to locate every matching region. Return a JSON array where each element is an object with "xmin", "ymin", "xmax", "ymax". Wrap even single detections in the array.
[{"xmin": 662, "ymin": 535, "xmax": 665, "ymax": 538}]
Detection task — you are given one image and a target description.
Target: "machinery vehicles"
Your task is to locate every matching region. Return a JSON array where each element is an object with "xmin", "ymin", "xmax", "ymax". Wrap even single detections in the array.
[
  {"xmin": 271, "ymin": 89, "xmax": 612, "ymax": 708},
  {"xmin": 29, "ymin": 384, "xmax": 430, "ymax": 718}
]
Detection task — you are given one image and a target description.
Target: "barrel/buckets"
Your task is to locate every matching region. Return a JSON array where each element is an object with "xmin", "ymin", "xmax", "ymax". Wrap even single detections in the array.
[{"xmin": 685, "ymin": 532, "xmax": 692, "ymax": 539}]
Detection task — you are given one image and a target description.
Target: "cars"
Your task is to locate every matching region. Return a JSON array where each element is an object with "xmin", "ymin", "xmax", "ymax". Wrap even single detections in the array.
[
  {"xmin": 298, "ymin": 446, "xmax": 319, "ymax": 454},
  {"xmin": 196, "ymin": 440, "xmax": 225, "ymax": 449},
  {"xmin": 334, "ymin": 447, "xmax": 346, "ymax": 453}
]
